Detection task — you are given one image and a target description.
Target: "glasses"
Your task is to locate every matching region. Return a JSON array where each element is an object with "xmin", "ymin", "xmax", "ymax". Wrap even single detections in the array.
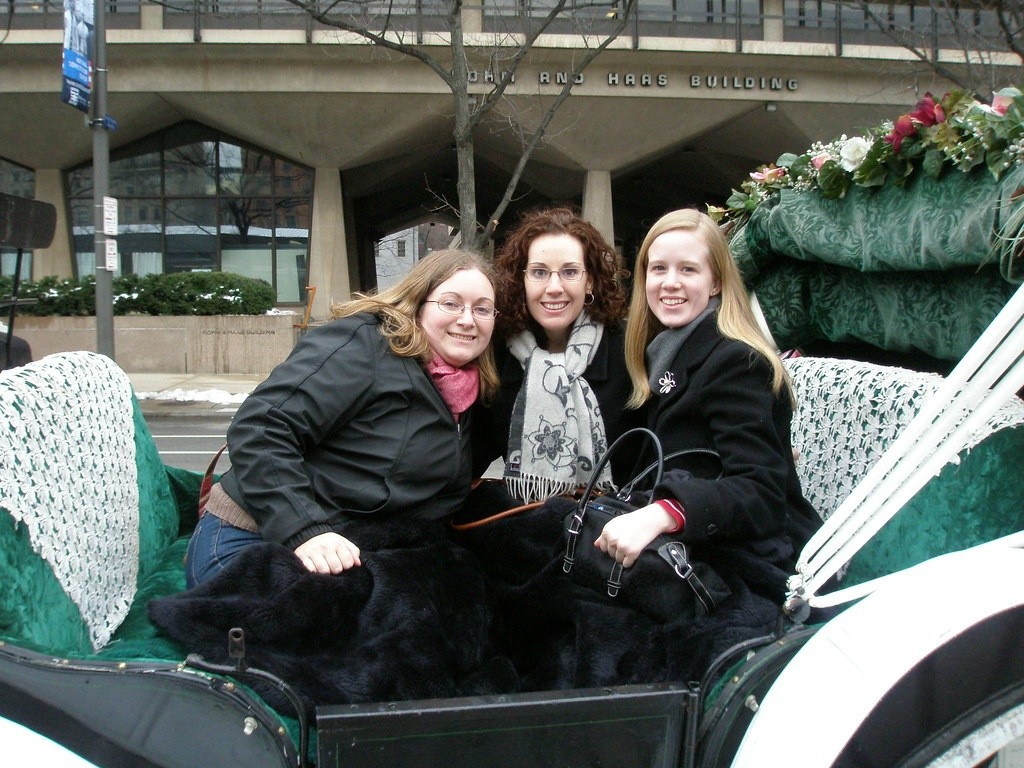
[
  {"xmin": 422, "ymin": 296, "xmax": 500, "ymax": 320},
  {"xmin": 523, "ymin": 266, "xmax": 591, "ymax": 282}
]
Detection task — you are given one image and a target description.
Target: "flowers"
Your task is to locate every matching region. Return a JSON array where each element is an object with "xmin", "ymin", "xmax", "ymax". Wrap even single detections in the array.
[{"xmin": 705, "ymin": 88, "xmax": 1024, "ymax": 223}]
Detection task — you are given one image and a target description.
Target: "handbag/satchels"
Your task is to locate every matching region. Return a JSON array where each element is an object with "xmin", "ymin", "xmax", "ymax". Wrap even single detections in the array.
[{"xmin": 561, "ymin": 426, "xmax": 733, "ymax": 620}]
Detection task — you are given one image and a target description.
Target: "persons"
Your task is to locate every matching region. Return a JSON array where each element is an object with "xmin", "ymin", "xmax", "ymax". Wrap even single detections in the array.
[
  {"xmin": 181, "ymin": 247, "xmax": 495, "ymax": 594},
  {"xmin": 594, "ymin": 208, "xmax": 801, "ymax": 568},
  {"xmin": 474, "ymin": 205, "xmax": 646, "ymax": 496}
]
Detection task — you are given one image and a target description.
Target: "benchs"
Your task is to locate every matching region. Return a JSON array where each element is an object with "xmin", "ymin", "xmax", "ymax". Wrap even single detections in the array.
[
  {"xmin": 0, "ymin": 350, "xmax": 316, "ymax": 768},
  {"xmin": 699, "ymin": 356, "xmax": 1024, "ymax": 768}
]
